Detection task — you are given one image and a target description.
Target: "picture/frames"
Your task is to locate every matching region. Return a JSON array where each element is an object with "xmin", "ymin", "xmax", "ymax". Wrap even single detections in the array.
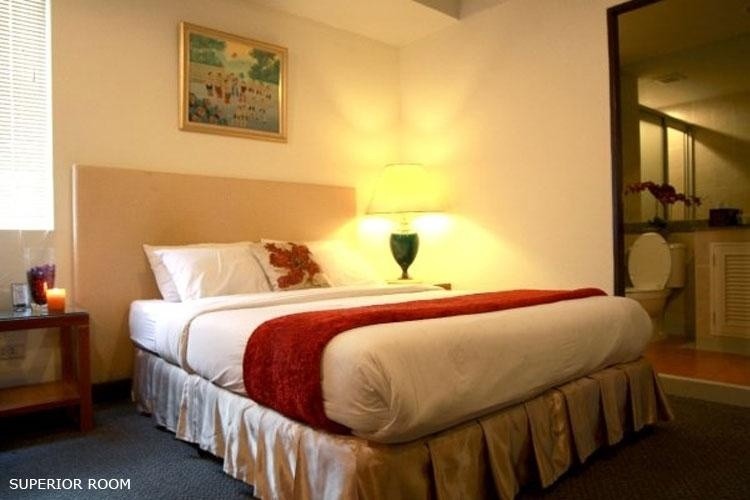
[{"xmin": 179, "ymin": 21, "xmax": 288, "ymax": 143}]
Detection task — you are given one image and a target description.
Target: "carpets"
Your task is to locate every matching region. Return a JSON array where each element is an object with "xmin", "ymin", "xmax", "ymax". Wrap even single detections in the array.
[{"xmin": 1, "ymin": 394, "xmax": 750, "ymax": 500}]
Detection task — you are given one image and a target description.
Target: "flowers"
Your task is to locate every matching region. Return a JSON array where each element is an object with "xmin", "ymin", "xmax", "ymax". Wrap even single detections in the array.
[
  {"xmin": 625, "ymin": 182, "xmax": 669, "ymax": 209},
  {"xmin": 669, "ymin": 186, "xmax": 702, "ymax": 207}
]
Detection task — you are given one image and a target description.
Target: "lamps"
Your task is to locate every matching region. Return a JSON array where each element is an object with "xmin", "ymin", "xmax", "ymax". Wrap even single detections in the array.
[{"xmin": 365, "ymin": 163, "xmax": 445, "ymax": 280}]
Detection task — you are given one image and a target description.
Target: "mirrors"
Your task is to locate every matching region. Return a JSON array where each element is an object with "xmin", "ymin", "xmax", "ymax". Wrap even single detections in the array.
[{"xmin": 620, "ymin": 33, "xmax": 750, "ymax": 224}]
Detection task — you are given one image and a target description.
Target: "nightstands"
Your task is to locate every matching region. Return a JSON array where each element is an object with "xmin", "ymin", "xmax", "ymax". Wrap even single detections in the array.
[{"xmin": 0, "ymin": 303, "xmax": 93, "ymax": 434}]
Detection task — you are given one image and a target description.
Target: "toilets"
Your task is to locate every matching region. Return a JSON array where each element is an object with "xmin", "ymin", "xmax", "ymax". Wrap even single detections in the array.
[{"xmin": 625, "ymin": 232, "xmax": 687, "ymax": 341}]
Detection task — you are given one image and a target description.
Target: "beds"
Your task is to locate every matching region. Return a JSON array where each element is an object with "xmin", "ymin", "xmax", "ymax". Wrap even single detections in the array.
[{"xmin": 128, "ymin": 286, "xmax": 674, "ymax": 500}]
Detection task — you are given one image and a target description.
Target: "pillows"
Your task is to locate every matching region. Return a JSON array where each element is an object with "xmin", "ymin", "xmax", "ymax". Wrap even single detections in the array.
[{"xmin": 143, "ymin": 241, "xmax": 374, "ymax": 301}]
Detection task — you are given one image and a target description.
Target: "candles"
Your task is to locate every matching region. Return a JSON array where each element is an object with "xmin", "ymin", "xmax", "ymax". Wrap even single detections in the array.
[{"xmin": 48, "ymin": 288, "xmax": 65, "ymax": 314}]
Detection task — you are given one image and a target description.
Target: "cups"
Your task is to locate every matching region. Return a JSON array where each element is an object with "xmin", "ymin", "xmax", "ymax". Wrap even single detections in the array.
[
  {"xmin": 12, "ymin": 282, "xmax": 28, "ymax": 312},
  {"xmin": 24, "ymin": 246, "xmax": 57, "ymax": 313},
  {"xmin": 46, "ymin": 287, "xmax": 66, "ymax": 313}
]
